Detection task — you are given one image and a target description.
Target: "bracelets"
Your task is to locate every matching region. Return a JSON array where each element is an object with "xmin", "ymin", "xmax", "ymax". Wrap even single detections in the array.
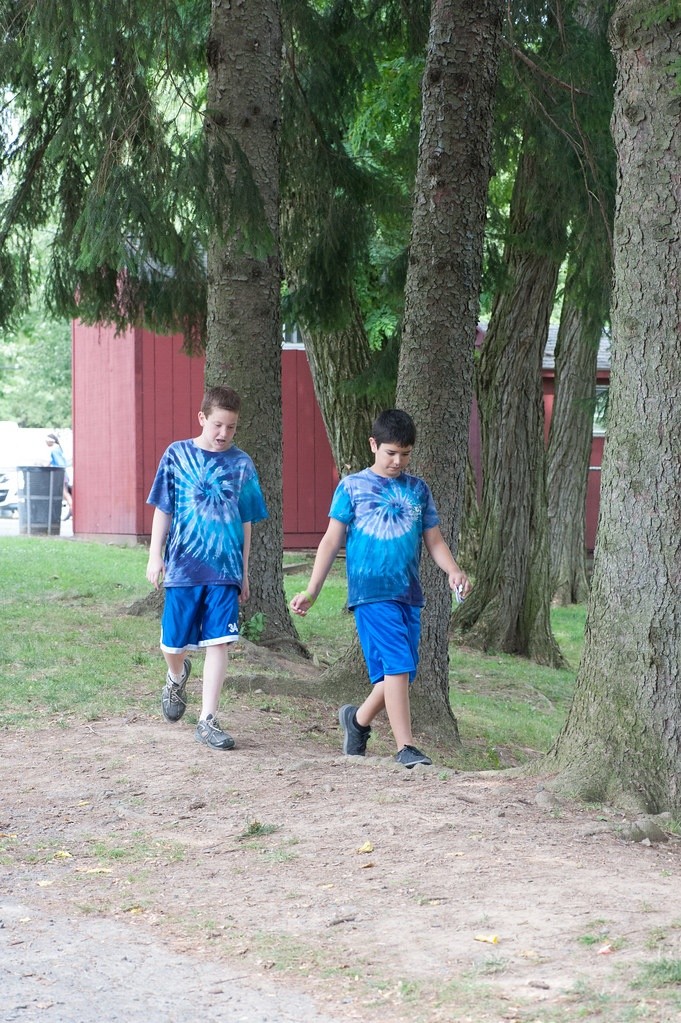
[{"xmin": 301, "ymin": 591, "xmax": 313, "ymax": 606}]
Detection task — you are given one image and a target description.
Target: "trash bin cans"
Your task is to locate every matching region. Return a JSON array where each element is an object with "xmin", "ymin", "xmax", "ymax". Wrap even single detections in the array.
[{"xmin": 15, "ymin": 466, "xmax": 65, "ymax": 536}]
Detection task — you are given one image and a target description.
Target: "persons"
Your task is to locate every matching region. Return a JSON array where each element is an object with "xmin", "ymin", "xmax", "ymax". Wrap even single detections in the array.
[
  {"xmin": 290, "ymin": 410, "xmax": 473, "ymax": 767},
  {"xmin": 145, "ymin": 387, "xmax": 270, "ymax": 749},
  {"xmin": 46, "ymin": 434, "xmax": 72, "ymax": 521}
]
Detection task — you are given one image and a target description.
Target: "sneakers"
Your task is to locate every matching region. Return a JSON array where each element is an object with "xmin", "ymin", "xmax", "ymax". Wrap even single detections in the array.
[
  {"xmin": 162, "ymin": 658, "xmax": 192, "ymax": 723},
  {"xmin": 338, "ymin": 704, "xmax": 371, "ymax": 757},
  {"xmin": 194, "ymin": 714, "xmax": 236, "ymax": 750},
  {"xmin": 396, "ymin": 744, "xmax": 432, "ymax": 768}
]
